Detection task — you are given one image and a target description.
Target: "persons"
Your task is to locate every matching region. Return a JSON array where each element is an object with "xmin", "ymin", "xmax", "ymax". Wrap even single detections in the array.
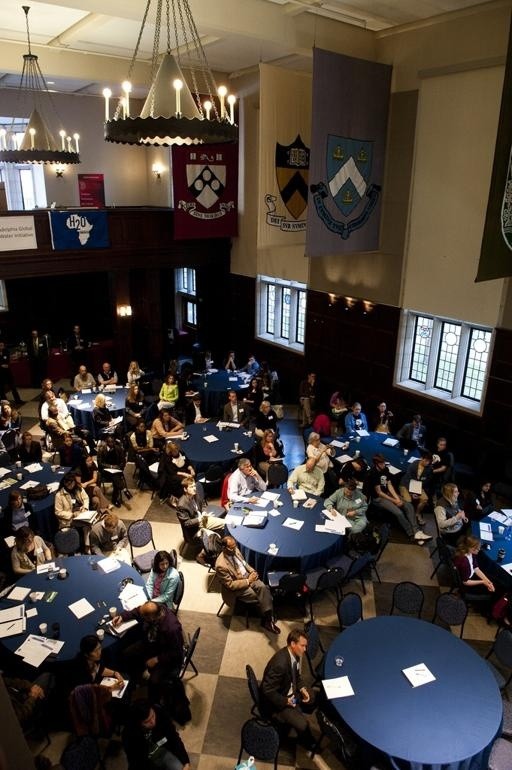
[
  {"xmin": 299, "ymin": 372, "xmax": 319, "ymax": 427},
  {"xmin": 330, "ymin": 391, "xmax": 496, "ymax": 620},
  {"xmin": 67, "ymin": 325, "xmax": 89, "ymax": 388},
  {"xmin": 95, "ymin": 349, "xmax": 278, "ymax": 430},
  {"xmin": 110, "ymin": 602, "xmax": 185, "ymax": 687},
  {"xmin": 1, "ymin": 662, "xmax": 67, "ymax": 733},
  {"xmin": 26, "ymin": 329, "xmax": 50, "ymax": 389},
  {"xmin": 0, "ymin": 398, "xmax": 180, "ymax": 610},
  {"xmin": 68, "ymin": 636, "xmax": 127, "ymax": 692},
  {"xmin": 259, "ymin": 629, "xmax": 327, "ymax": 756},
  {"xmin": 122, "ymin": 696, "xmax": 190, "ymax": 770},
  {"xmin": 164, "ymin": 426, "xmax": 370, "ymax": 634}
]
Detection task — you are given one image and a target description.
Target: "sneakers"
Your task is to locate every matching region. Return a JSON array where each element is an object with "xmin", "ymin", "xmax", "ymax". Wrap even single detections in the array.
[
  {"xmin": 111, "ymin": 498, "xmax": 121, "ymax": 508},
  {"xmin": 409, "ymin": 530, "xmax": 433, "ymax": 546},
  {"xmin": 124, "ymin": 490, "xmax": 133, "ymax": 500},
  {"xmin": 416, "ymin": 514, "xmax": 426, "ymax": 526}
]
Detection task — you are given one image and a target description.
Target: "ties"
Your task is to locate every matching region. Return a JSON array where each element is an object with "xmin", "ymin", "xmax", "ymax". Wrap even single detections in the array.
[{"xmin": 292, "ymin": 662, "xmax": 299, "ymax": 696}]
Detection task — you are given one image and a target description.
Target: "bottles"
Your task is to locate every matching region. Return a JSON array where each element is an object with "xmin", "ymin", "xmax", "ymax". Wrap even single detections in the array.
[
  {"xmin": 497, "ymin": 549, "xmax": 505, "ymax": 561},
  {"xmin": 90, "ymin": 556, "xmax": 98, "ymax": 575},
  {"xmin": 48, "ymin": 564, "xmax": 55, "ymax": 579}
]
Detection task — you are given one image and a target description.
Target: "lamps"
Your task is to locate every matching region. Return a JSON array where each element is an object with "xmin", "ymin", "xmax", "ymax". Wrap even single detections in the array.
[
  {"xmin": 1, "ymin": 1, "xmax": 84, "ymax": 165},
  {"xmin": 54, "ymin": 164, "xmax": 65, "ymax": 178},
  {"xmin": 103, "ymin": 0, "xmax": 242, "ymax": 147},
  {"xmin": 151, "ymin": 163, "xmax": 161, "ymax": 178}
]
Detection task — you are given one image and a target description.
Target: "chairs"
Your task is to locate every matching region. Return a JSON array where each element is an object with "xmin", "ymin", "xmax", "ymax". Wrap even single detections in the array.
[{"xmin": 0, "ymin": 361, "xmax": 510, "ymax": 770}]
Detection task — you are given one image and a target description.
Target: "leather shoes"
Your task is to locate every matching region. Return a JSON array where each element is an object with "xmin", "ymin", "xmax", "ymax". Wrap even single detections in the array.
[{"xmin": 260, "ymin": 615, "xmax": 281, "ymax": 634}]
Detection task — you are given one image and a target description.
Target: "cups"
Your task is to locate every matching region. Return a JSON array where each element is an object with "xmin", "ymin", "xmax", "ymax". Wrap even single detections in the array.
[
  {"xmin": 269, "ymin": 543, "xmax": 276, "ymax": 552},
  {"xmin": 202, "ymin": 423, "xmax": 253, "ymax": 451},
  {"xmin": 346, "ymin": 436, "xmax": 408, "ymax": 457},
  {"xmin": 59, "ymin": 568, "xmax": 67, "ymax": 578},
  {"xmin": 29, "ymin": 592, "xmax": 117, "ymax": 640},
  {"xmin": 274, "ymin": 501, "xmax": 279, "ymax": 508},
  {"xmin": 15, "ymin": 460, "xmax": 55, "ymax": 480},
  {"xmin": 498, "ymin": 526, "xmax": 505, "ymax": 535},
  {"xmin": 293, "ymin": 500, "xmax": 299, "ymax": 509},
  {"xmin": 335, "ymin": 656, "xmax": 344, "ymax": 666}
]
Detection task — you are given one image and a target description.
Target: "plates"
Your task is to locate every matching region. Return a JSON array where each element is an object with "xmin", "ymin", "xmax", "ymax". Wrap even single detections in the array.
[{"xmin": 57, "ymin": 572, "xmax": 69, "ymax": 580}]
